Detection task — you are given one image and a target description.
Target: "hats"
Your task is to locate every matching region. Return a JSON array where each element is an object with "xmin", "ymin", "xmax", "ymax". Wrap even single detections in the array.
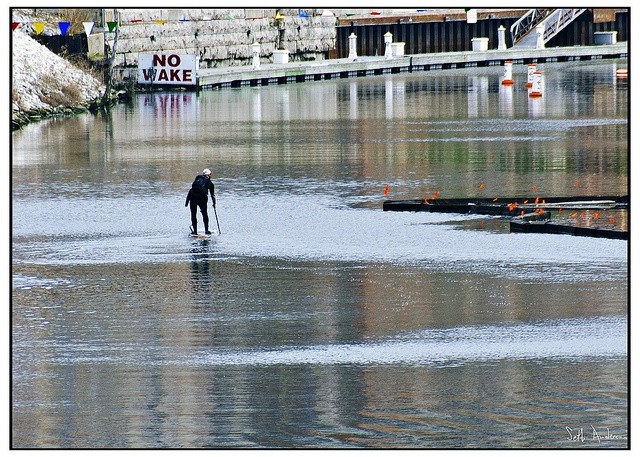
[{"xmin": 203, "ymin": 169, "xmax": 212, "ymax": 175}]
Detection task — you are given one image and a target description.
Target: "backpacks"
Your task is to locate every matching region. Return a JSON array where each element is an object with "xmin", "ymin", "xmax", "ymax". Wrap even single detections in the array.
[{"xmin": 192, "ymin": 175, "xmax": 210, "ymax": 194}]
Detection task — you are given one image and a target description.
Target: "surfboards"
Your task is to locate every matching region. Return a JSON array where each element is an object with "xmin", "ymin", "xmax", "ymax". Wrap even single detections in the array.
[{"xmin": 189, "ymin": 228, "xmax": 218, "ymax": 238}]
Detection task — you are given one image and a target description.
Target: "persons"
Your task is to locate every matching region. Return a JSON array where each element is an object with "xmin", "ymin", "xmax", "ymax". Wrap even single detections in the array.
[{"xmin": 184, "ymin": 169, "xmax": 217, "ymax": 235}]
